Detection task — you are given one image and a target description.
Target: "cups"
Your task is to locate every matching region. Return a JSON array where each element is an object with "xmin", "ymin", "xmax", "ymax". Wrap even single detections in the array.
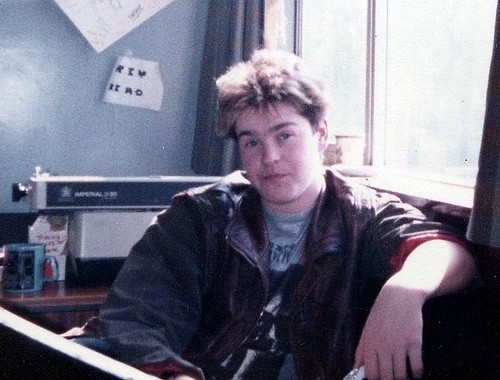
[{"xmin": 3, "ymin": 243, "xmax": 59, "ymax": 293}]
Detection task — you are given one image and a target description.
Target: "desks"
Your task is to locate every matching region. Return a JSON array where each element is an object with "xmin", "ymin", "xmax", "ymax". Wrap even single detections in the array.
[{"xmin": 0, "ymin": 280, "xmax": 109, "ymax": 335}]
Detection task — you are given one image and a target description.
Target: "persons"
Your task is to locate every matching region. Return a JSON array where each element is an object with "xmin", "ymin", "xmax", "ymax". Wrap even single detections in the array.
[{"xmin": 69, "ymin": 50, "xmax": 479, "ymax": 380}]
